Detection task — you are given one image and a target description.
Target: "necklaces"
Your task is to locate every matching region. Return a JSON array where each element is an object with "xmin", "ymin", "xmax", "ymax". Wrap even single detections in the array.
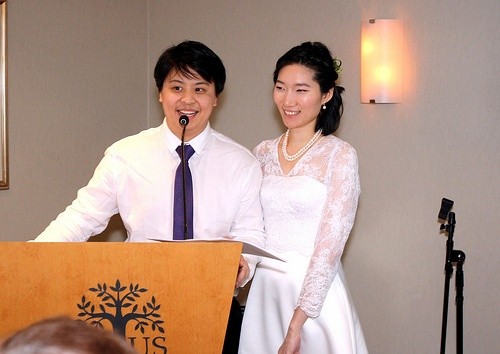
[{"xmin": 283, "ymin": 128, "xmax": 323, "ymax": 161}]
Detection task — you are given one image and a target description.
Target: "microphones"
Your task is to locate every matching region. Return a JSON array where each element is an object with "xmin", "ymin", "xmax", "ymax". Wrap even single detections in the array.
[{"xmin": 179, "ymin": 115, "xmax": 190, "ymax": 240}]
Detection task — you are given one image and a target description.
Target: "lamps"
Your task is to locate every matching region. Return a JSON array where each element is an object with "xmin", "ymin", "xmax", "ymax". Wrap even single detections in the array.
[{"xmin": 360, "ymin": 19, "xmax": 412, "ymax": 103}]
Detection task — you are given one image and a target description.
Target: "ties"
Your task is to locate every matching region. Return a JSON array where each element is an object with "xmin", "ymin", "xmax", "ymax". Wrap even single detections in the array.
[{"xmin": 173, "ymin": 145, "xmax": 195, "ymax": 240}]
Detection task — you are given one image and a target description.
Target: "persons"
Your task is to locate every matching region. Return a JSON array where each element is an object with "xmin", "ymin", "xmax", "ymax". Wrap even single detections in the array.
[
  {"xmin": 26, "ymin": 40, "xmax": 265, "ymax": 354},
  {"xmin": 0, "ymin": 316, "xmax": 136, "ymax": 354},
  {"xmin": 236, "ymin": 41, "xmax": 370, "ymax": 354}
]
